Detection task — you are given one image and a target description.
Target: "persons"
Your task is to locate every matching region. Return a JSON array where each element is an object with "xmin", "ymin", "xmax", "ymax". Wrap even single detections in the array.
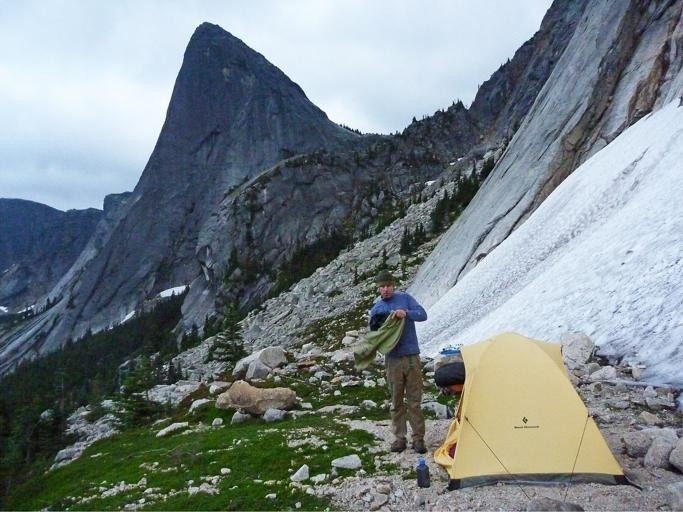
[
  {"xmin": 369, "ymin": 269, "xmax": 427, "ymax": 454},
  {"xmin": 436, "ymin": 362, "xmax": 465, "ymax": 393}
]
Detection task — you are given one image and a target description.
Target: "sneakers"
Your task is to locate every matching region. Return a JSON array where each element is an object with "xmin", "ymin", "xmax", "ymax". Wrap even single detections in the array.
[
  {"xmin": 412, "ymin": 439, "xmax": 427, "ymax": 453},
  {"xmin": 391, "ymin": 440, "xmax": 406, "ymax": 451}
]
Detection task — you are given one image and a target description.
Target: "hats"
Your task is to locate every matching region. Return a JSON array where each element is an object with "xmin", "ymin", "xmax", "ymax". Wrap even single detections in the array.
[{"xmin": 376, "ymin": 269, "xmax": 393, "ymax": 288}]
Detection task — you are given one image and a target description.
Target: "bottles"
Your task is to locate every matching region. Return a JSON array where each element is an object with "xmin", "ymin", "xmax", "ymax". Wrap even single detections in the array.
[{"xmin": 415, "ymin": 457, "xmax": 430, "ymax": 488}]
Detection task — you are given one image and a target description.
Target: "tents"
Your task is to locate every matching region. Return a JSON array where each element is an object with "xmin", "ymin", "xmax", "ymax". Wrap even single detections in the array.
[{"xmin": 435, "ymin": 331, "xmax": 642, "ymax": 491}]
